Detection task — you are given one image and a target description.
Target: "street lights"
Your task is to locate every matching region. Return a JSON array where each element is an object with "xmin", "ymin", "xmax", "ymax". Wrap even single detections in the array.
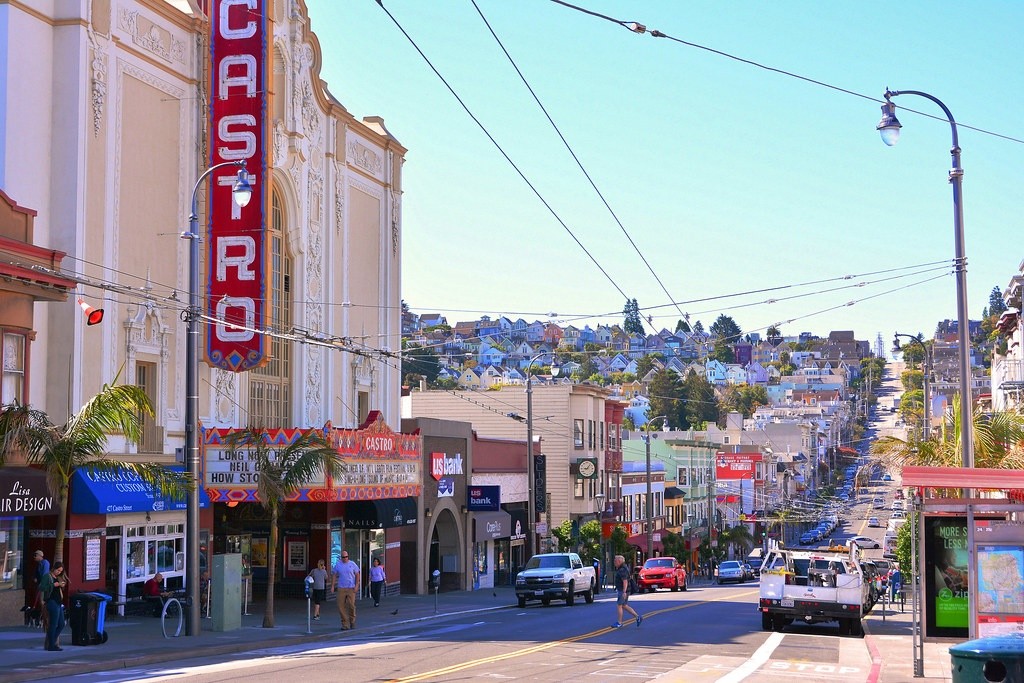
[
  {"xmin": 180, "ymin": 160, "xmax": 254, "ymax": 634},
  {"xmin": 644, "ymin": 417, "xmax": 671, "ymax": 560},
  {"xmin": 595, "ymin": 493, "xmax": 606, "ymax": 592},
  {"xmin": 876, "ymin": 86, "xmax": 975, "ymax": 500},
  {"xmin": 889, "ymin": 330, "xmax": 930, "ymax": 443},
  {"xmin": 706, "ymin": 454, "xmax": 725, "ymax": 580},
  {"xmin": 526, "ymin": 351, "xmax": 562, "ymax": 552},
  {"xmin": 740, "ymin": 471, "xmax": 755, "ymax": 561}
]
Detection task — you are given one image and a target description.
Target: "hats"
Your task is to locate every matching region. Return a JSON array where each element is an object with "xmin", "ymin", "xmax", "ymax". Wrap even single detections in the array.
[{"xmin": 35, "ymin": 550, "xmax": 44, "ymax": 557}]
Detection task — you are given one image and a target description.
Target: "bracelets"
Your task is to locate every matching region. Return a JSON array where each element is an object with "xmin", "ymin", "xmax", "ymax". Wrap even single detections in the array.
[{"xmin": 385, "ymin": 581, "xmax": 386, "ymax": 582}]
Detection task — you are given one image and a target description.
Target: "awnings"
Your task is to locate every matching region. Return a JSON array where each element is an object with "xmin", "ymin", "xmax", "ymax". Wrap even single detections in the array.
[
  {"xmin": 345, "ymin": 497, "xmax": 417, "ymax": 530},
  {"xmin": 626, "ymin": 529, "xmax": 672, "ymax": 553},
  {"xmin": 0, "ymin": 466, "xmax": 61, "ymax": 516},
  {"xmin": 71, "ymin": 466, "xmax": 209, "ymax": 514}
]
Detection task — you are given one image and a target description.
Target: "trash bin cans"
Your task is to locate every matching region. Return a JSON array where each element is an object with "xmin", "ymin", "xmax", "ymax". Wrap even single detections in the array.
[
  {"xmin": 892, "ymin": 571, "xmax": 901, "ymax": 603},
  {"xmin": 69, "ymin": 593, "xmax": 106, "ymax": 646},
  {"xmin": 88, "ymin": 592, "xmax": 113, "ymax": 644},
  {"xmin": 948, "ymin": 633, "xmax": 1024, "ymax": 683}
]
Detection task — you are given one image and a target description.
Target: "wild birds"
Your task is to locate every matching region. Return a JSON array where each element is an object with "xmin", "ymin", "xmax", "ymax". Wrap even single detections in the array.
[
  {"xmin": 389, "ymin": 609, "xmax": 399, "ymax": 615},
  {"xmin": 493, "ymin": 592, "xmax": 496, "ymax": 598}
]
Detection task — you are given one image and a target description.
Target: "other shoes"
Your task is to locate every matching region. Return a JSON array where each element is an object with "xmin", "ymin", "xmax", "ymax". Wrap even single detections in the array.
[
  {"xmin": 374, "ymin": 601, "xmax": 379, "ymax": 607},
  {"xmin": 341, "ymin": 626, "xmax": 348, "ymax": 630},
  {"xmin": 48, "ymin": 645, "xmax": 63, "ymax": 651},
  {"xmin": 351, "ymin": 625, "xmax": 356, "ymax": 629},
  {"xmin": 313, "ymin": 615, "xmax": 320, "ymax": 620}
]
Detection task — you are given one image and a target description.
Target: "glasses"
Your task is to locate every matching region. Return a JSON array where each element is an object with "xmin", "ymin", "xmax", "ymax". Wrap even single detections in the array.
[{"xmin": 342, "ymin": 556, "xmax": 348, "ymax": 559}]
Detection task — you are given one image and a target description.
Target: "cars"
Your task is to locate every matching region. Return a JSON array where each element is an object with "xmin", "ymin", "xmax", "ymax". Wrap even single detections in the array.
[
  {"xmin": 883, "ymin": 475, "xmax": 891, "ymax": 481},
  {"xmin": 744, "ymin": 564, "xmax": 755, "ymax": 580},
  {"xmin": 799, "ymin": 397, "xmax": 906, "ymax": 597},
  {"xmin": 845, "ymin": 536, "xmax": 880, "ymax": 550},
  {"xmin": 636, "ymin": 556, "xmax": 688, "ymax": 592},
  {"xmin": 868, "ymin": 517, "xmax": 881, "ymax": 527},
  {"xmin": 718, "ymin": 560, "xmax": 745, "ymax": 584}
]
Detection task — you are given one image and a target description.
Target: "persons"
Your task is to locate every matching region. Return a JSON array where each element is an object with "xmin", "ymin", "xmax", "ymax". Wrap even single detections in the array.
[
  {"xmin": 308, "ymin": 559, "xmax": 328, "ymax": 621},
  {"xmin": 144, "ymin": 574, "xmax": 173, "ymax": 618},
  {"xmin": 331, "ymin": 551, "xmax": 359, "ymax": 630},
  {"xmin": 892, "ymin": 563, "xmax": 906, "ymax": 604},
  {"xmin": 25, "ymin": 550, "xmax": 66, "ymax": 651},
  {"xmin": 200, "ymin": 571, "xmax": 210, "ymax": 613},
  {"xmin": 369, "ymin": 558, "xmax": 387, "ymax": 607}
]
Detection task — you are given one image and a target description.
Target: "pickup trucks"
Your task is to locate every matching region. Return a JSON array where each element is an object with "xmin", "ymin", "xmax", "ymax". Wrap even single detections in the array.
[{"xmin": 515, "ymin": 553, "xmax": 597, "ymax": 607}]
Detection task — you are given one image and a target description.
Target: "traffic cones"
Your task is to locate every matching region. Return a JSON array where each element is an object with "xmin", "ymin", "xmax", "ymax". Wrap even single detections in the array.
[{"xmin": 77, "ymin": 298, "xmax": 105, "ymax": 326}]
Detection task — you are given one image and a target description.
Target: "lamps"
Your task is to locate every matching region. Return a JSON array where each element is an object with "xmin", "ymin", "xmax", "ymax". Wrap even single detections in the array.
[
  {"xmin": 426, "ymin": 508, "xmax": 433, "ymax": 517},
  {"xmin": 462, "ymin": 505, "xmax": 467, "ymax": 514},
  {"xmin": 146, "ymin": 512, "xmax": 151, "ymax": 520}
]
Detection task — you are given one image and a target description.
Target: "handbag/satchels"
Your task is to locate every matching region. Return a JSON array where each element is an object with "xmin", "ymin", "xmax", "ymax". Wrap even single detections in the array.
[{"xmin": 630, "ymin": 579, "xmax": 639, "ymax": 595}]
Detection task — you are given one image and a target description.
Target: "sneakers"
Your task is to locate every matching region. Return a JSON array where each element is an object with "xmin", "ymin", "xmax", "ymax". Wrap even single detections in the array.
[
  {"xmin": 611, "ymin": 622, "xmax": 623, "ymax": 628},
  {"xmin": 637, "ymin": 615, "xmax": 642, "ymax": 627}
]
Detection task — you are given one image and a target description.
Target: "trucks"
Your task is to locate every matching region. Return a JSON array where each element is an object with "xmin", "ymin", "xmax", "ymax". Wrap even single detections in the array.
[{"xmin": 752, "ymin": 538, "xmax": 871, "ymax": 636}]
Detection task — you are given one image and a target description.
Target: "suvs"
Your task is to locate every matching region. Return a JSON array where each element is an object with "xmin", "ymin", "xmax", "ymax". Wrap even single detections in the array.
[{"xmin": 747, "ymin": 559, "xmax": 765, "ymax": 576}]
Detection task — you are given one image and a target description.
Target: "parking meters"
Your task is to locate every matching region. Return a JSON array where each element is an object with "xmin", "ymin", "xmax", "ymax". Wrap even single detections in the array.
[
  {"xmin": 302, "ymin": 575, "xmax": 314, "ymax": 632},
  {"xmin": 431, "ymin": 570, "xmax": 441, "ymax": 616}
]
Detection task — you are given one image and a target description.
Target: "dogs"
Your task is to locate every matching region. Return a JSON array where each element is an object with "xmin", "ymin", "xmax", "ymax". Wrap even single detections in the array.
[{"xmin": 20, "ymin": 604, "xmax": 42, "ymax": 628}]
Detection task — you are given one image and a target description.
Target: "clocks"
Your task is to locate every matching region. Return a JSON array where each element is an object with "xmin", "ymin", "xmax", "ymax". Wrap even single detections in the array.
[{"xmin": 576, "ymin": 457, "xmax": 599, "ymax": 479}]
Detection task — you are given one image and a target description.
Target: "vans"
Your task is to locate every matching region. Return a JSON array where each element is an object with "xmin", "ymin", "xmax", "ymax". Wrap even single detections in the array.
[{"xmin": 872, "ymin": 498, "xmax": 885, "ymax": 509}]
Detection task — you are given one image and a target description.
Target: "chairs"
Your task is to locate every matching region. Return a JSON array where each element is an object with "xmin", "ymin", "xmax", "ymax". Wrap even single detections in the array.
[{"xmin": 141, "ymin": 594, "xmax": 172, "ymax": 617}]
